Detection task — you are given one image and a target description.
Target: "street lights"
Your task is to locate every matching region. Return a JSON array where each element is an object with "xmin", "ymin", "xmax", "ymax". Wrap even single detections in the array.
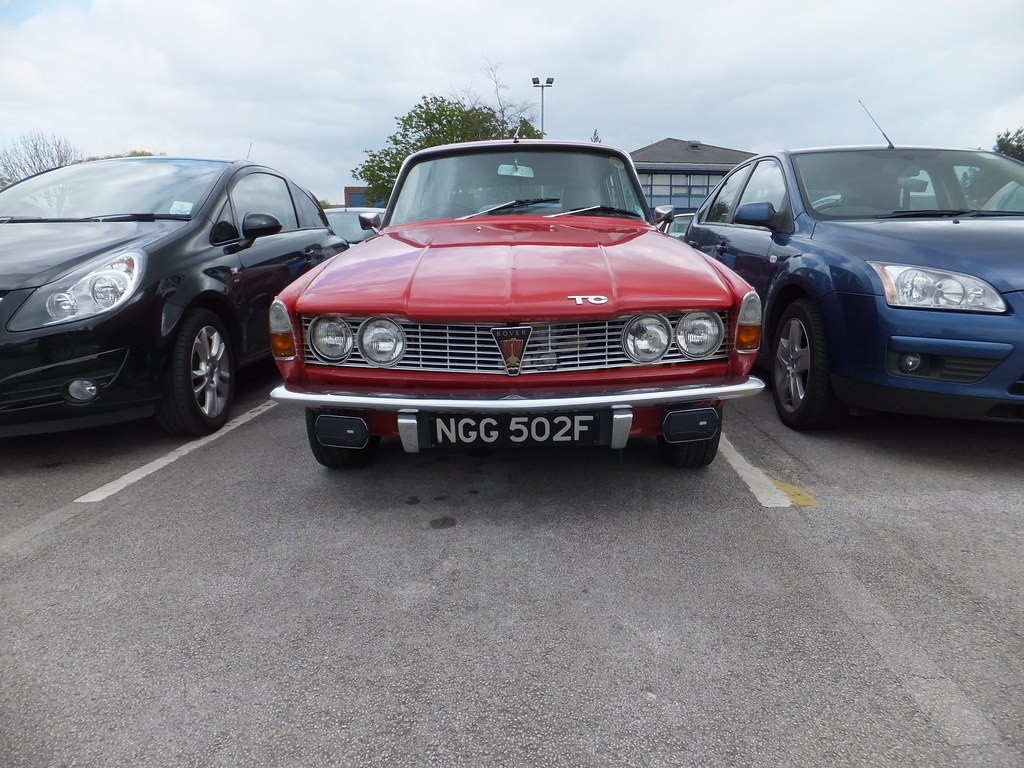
[{"xmin": 532, "ymin": 76, "xmax": 555, "ymax": 142}]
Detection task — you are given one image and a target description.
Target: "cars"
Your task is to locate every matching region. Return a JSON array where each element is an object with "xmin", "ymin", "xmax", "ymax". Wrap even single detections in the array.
[
  {"xmin": 691, "ymin": 144, "xmax": 1024, "ymax": 432},
  {"xmin": 659, "ymin": 213, "xmax": 700, "ymax": 241},
  {"xmin": 0, "ymin": 158, "xmax": 351, "ymax": 441},
  {"xmin": 322, "ymin": 206, "xmax": 392, "ymax": 248},
  {"xmin": 808, "ymin": 182, "xmax": 1024, "ymax": 215},
  {"xmin": 272, "ymin": 137, "xmax": 773, "ymax": 467}
]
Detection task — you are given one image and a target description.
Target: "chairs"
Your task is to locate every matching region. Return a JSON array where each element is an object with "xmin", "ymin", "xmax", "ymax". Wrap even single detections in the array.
[{"xmin": 562, "ymin": 179, "xmax": 601, "ymax": 212}]
